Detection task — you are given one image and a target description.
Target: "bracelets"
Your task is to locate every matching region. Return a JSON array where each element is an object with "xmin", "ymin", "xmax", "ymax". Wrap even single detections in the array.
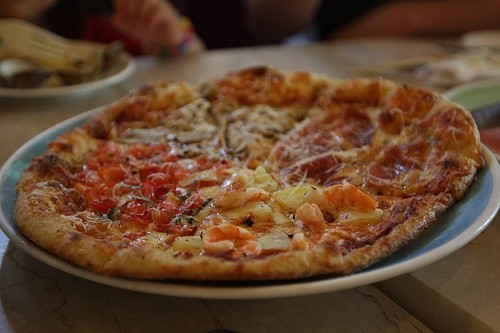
[{"xmin": 151, "ymin": 18, "xmax": 193, "ymax": 59}]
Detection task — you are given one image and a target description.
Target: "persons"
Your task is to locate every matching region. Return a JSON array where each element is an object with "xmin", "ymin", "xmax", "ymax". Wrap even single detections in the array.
[
  {"xmin": 302, "ymin": 0, "xmax": 500, "ymax": 43},
  {"xmin": 0, "ymin": 0, "xmax": 206, "ymax": 60}
]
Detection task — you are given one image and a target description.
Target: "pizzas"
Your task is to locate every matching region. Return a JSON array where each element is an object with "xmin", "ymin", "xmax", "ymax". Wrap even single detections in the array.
[{"xmin": 14, "ymin": 63, "xmax": 483, "ymax": 279}]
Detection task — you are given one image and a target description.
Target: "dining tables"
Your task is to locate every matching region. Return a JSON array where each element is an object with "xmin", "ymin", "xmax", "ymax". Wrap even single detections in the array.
[{"xmin": 0, "ymin": 34, "xmax": 500, "ymax": 332}]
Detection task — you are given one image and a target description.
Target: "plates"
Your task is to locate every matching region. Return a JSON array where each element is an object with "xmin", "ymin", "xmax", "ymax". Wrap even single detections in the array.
[
  {"xmin": 1, "ymin": 102, "xmax": 500, "ymax": 299},
  {"xmin": 441, "ymin": 79, "xmax": 500, "ymax": 116},
  {"xmin": 0, "ymin": 52, "xmax": 136, "ymax": 99}
]
